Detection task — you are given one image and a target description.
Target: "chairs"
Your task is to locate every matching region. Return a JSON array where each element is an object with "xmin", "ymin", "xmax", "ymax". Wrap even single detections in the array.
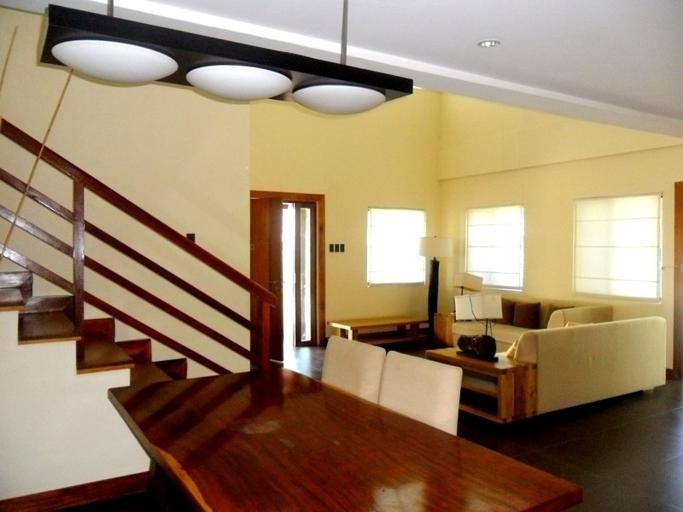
[
  {"xmin": 319, "ymin": 338, "xmax": 387, "ymax": 409},
  {"xmin": 377, "ymin": 348, "xmax": 463, "ymax": 438}
]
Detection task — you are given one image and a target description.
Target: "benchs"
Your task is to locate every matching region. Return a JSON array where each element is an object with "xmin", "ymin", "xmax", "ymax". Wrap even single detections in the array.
[{"xmin": 324, "ymin": 311, "xmax": 428, "ymax": 343}]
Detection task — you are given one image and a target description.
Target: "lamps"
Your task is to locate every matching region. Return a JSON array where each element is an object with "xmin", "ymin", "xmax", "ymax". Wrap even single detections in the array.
[
  {"xmin": 453, "ymin": 293, "xmax": 504, "ymax": 364},
  {"xmin": 416, "ymin": 233, "xmax": 454, "ymax": 349},
  {"xmin": 33, "ymin": 4, "xmax": 412, "ymax": 115},
  {"xmin": 452, "ymin": 271, "xmax": 484, "ymax": 295}
]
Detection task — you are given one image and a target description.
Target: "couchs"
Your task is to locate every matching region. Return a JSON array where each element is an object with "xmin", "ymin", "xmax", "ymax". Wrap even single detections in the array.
[
  {"xmin": 432, "ymin": 289, "xmax": 613, "ymax": 358},
  {"xmin": 500, "ymin": 314, "xmax": 667, "ymax": 420}
]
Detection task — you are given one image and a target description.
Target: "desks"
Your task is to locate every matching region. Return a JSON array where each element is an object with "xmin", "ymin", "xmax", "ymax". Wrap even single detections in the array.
[{"xmin": 107, "ymin": 364, "xmax": 584, "ymax": 512}]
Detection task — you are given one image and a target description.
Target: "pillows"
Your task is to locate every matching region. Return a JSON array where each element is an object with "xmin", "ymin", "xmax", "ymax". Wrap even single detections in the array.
[{"xmin": 492, "ymin": 296, "xmax": 575, "ymax": 329}]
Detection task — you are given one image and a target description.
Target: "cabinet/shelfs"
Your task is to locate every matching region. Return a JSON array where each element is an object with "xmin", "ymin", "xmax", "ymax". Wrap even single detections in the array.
[{"xmin": 420, "ymin": 346, "xmax": 514, "ymax": 425}]
